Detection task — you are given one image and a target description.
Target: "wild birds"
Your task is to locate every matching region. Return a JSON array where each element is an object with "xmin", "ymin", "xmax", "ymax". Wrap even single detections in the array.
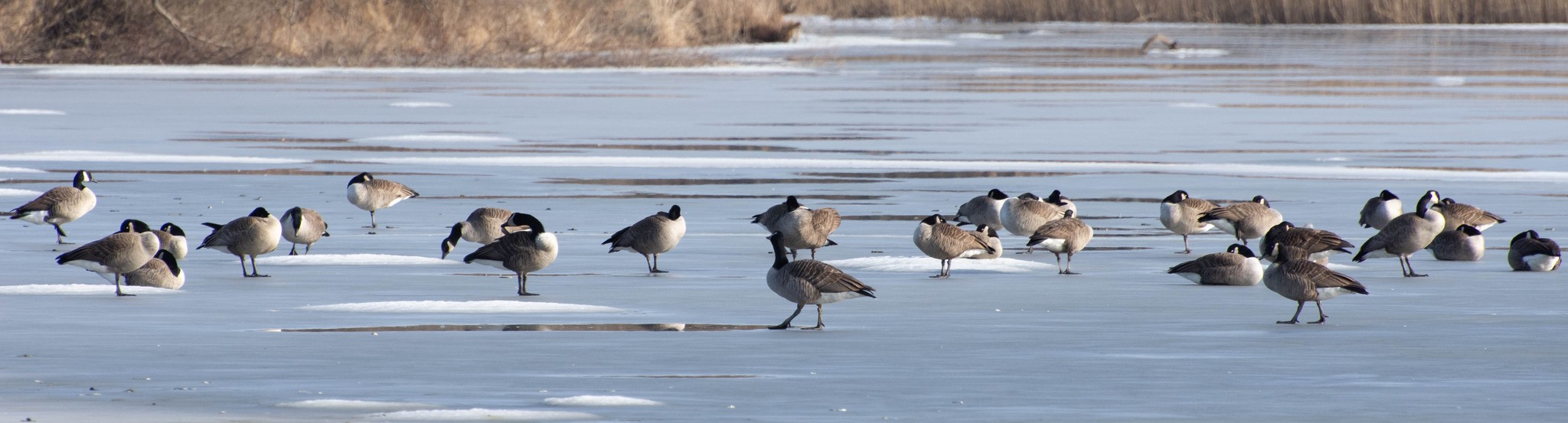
[
  {"xmin": 1352, "ymin": 189, "xmax": 1563, "ymax": 277},
  {"xmin": 1, "ymin": 169, "xmax": 330, "ymax": 295},
  {"xmin": 752, "ymin": 187, "xmax": 1371, "ymax": 328},
  {"xmin": 346, "ymin": 172, "xmax": 687, "ymax": 294}
]
